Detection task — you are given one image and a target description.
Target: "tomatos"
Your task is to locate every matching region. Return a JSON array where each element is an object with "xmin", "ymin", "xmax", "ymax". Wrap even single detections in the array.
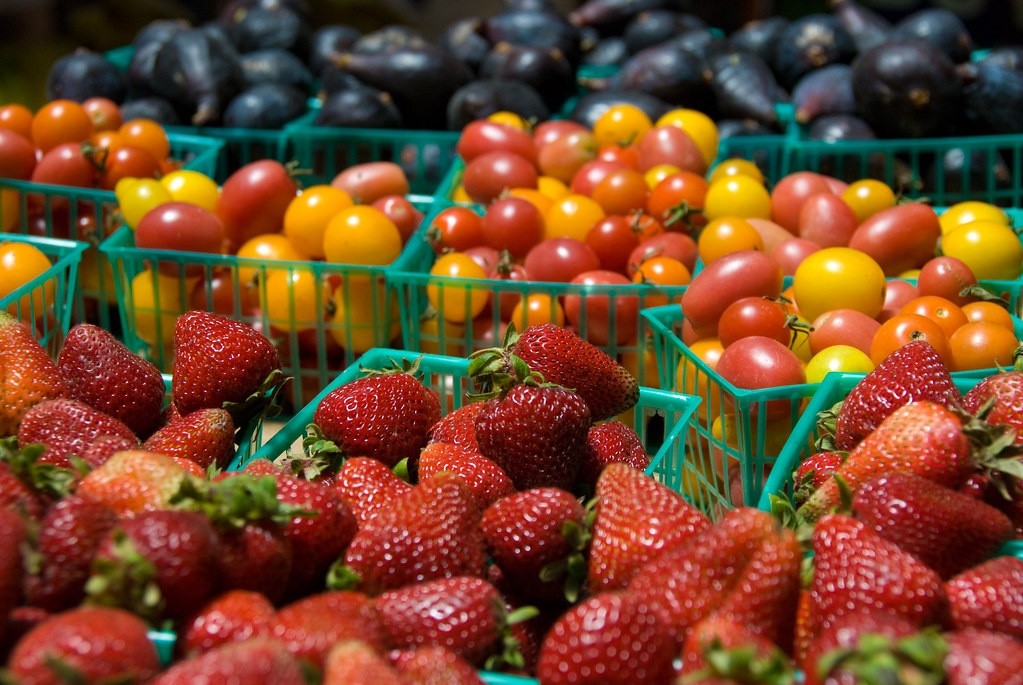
[
  {"xmin": 677, "ymin": 159, "xmax": 1023, "ymax": 517},
  {"xmin": 0, "ymin": 99, "xmax": 721, "ymax": 387}
]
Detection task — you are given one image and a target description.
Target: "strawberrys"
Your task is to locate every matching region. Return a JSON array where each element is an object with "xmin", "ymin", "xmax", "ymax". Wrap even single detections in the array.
[{"xmin": 0, "ymin": 310, "xmax": 1023, "ymax": 685}]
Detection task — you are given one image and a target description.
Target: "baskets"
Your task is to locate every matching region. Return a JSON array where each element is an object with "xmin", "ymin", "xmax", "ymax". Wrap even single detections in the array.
[
  {"xmin": 234, "ymin": 339, "xmax": 702, "ymax": 499},
  {"xmin": 0, "ymin": 27, "xmax": 1022, "ymax": 361},
  {"xmin": 642, "ymin": 303, "xmax": 1023, "ymax": 528},
  {"xmin": 140, "ymin": 627, "xmax": 546, "ymax": 683},
  {"xmin": 748, "ymin": 362, "xmax": 1023, "ymax": 568},
  {"xmin": 156, "ymin": 369, "xmax": 279, "ymax": 479}
]
